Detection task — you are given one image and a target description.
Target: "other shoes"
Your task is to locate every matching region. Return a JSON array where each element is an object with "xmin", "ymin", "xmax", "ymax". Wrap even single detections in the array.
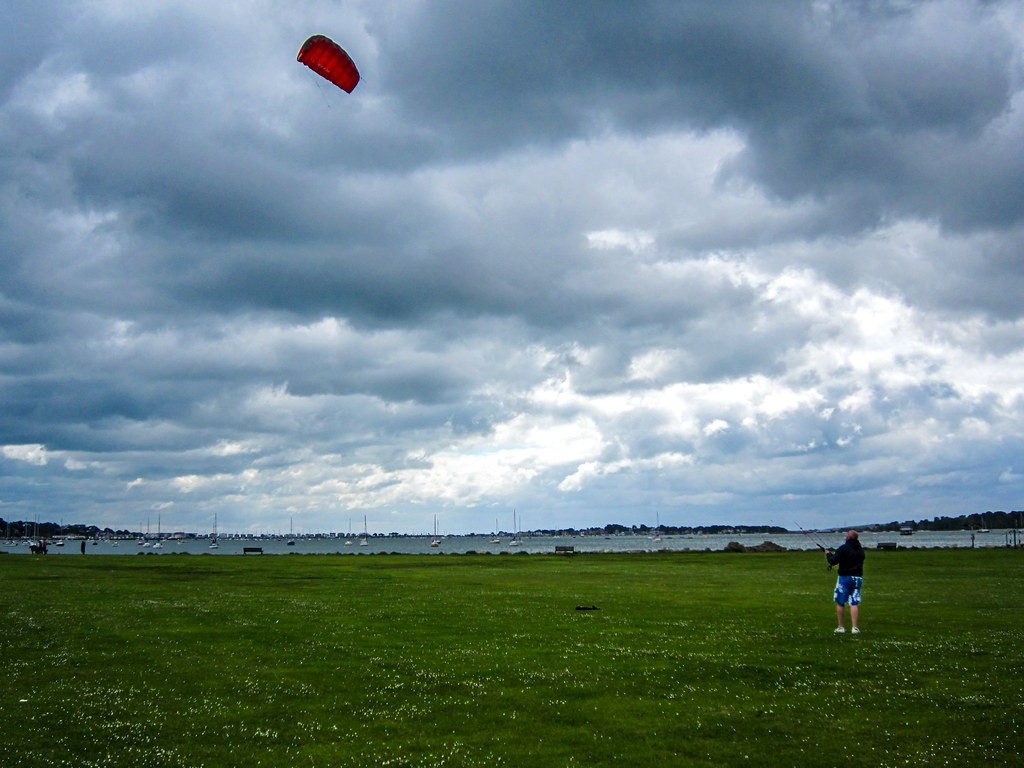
[
  {"xmin": 851, "ymin": 627, "xmax": 860, "ymax": 634},
  {"xmin": 835, "ymin": 627, "xmax": 845, "ymax": 633}
]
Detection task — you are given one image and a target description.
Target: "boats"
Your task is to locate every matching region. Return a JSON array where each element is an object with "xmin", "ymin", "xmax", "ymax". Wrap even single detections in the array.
[{"xmin": 6, "ymin": 517, "xmax": 996, "ymax": 546}]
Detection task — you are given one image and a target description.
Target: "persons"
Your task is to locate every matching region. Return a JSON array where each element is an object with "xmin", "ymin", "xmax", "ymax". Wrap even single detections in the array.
[{"xmin": 824, "ymin": 530, "xmax": 865, "ymax": 634}]
